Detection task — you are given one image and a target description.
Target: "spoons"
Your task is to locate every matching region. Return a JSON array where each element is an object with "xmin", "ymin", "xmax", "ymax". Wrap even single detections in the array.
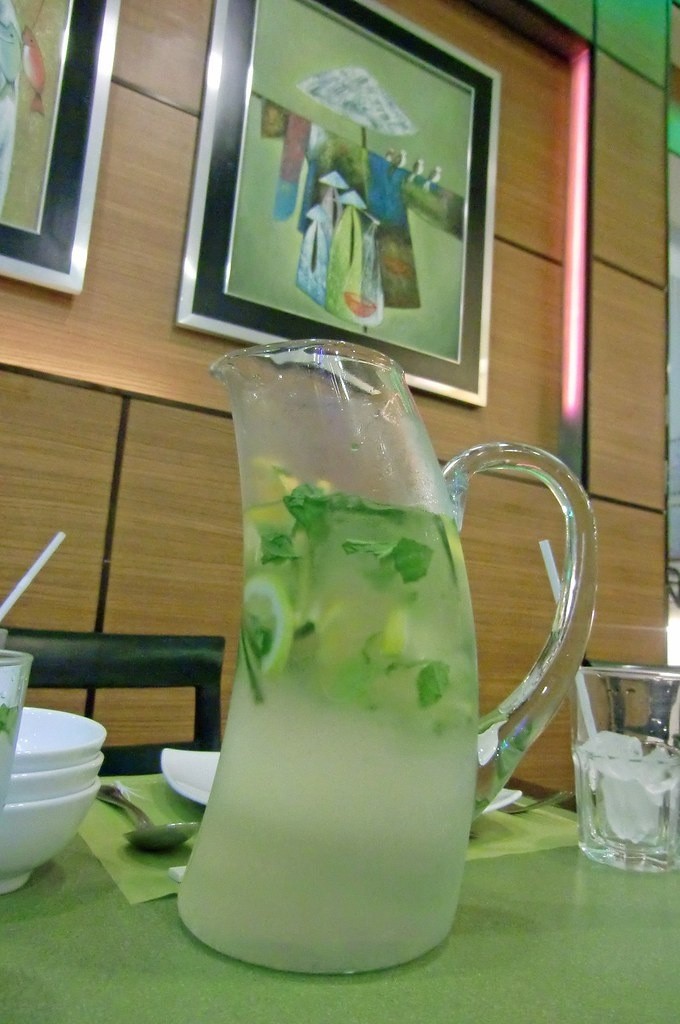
[{"xmin": 95, "ymin": 784, "xmax": 198, "ymax": 851}]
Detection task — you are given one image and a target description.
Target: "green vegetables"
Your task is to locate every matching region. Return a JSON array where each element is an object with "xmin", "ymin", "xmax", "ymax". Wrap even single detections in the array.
[{"xmin": 236, "ymin": 485, "xmax": 469, "ymax": 737}]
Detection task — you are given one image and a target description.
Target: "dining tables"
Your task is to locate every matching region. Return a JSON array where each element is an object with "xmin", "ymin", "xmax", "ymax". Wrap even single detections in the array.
[{"xmin": 1, "ymin": 773, "xmax": 680, "ymax": 1024}]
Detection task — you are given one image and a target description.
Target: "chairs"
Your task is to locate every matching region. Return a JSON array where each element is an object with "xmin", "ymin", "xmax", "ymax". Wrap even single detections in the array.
[{"xmin": 1, "ymin": 627, "xmax": 226, "ymax": 778}]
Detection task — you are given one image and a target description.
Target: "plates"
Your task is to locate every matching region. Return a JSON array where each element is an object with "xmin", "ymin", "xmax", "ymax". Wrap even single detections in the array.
[{"xmin": 162, "ymin": 748, "xmax": 523, "ymax": 816}]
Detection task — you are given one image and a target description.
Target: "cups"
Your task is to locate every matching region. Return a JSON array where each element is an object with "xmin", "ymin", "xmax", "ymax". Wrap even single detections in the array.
[
  {"xmin": 178, "ymin": 339, "xmax": 599, "ymax": 976},
  {"xmin": 569, "ymin": 662, "xmax": 680, "ymax": 874},
  {"xmin": 0, "ymin": 649, "xmax": 33, "ymax": 839}
]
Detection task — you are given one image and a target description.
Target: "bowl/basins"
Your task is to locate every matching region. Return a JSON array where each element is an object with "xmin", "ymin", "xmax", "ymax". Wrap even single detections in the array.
[{"xmin": 0, "ymin": 706, "xmax": 107, "ymax": 897}]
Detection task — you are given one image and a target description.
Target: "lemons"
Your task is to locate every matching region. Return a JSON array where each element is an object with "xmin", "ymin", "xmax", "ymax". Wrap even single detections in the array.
[{"xmin": 239, "ymin": 457, "xmax": 408, "ymax": 680}]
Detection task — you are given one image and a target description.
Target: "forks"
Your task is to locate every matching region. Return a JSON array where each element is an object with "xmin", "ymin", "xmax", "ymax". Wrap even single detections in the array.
[{"xmin": 494, "ymin": 790, "xmax": 574, "ymax": 815}]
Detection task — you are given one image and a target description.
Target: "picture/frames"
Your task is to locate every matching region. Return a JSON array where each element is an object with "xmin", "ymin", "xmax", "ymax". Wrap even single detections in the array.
[
  {"xmin": 0, "ymin": 0, "xmax": 122, "ymax": 296},
  {"xmin": 174, "ymin": 1, "xmax": 504, "ymax": 407}
]
